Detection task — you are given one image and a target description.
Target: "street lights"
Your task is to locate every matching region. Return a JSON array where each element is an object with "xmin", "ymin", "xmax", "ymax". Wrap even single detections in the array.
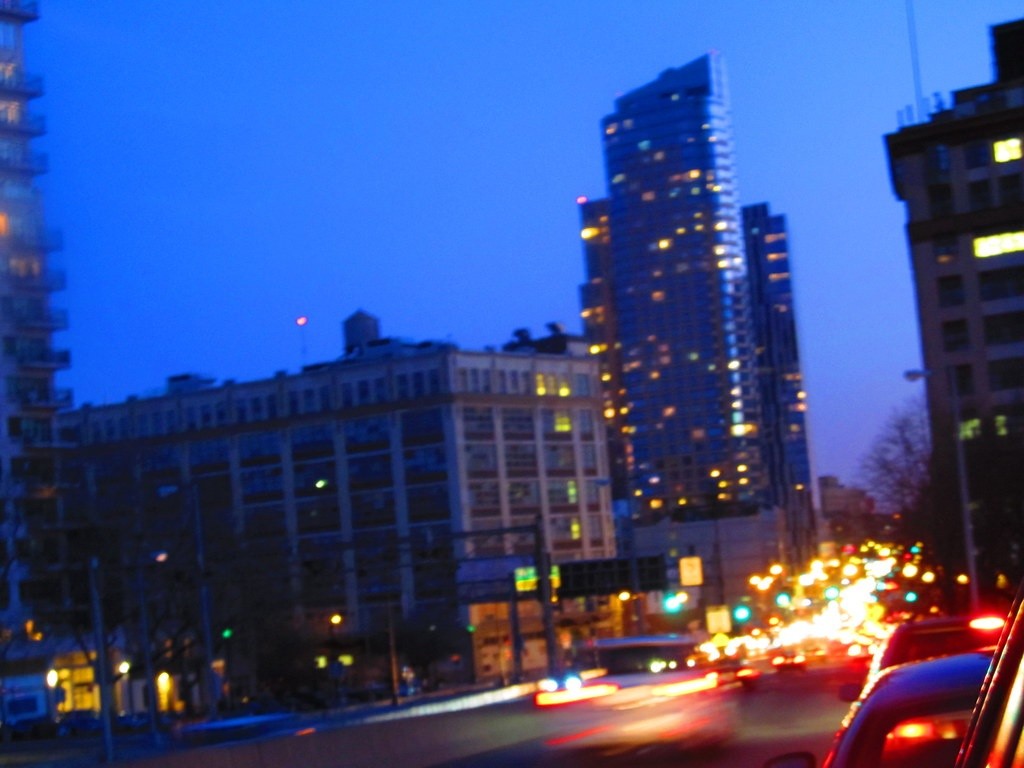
[
  {"xmin": 0, "ymin": 456, "xmax": 660, "ymax": 768},
  {"xmin": 905, "ymin": 357, "xmax": 983, "ymax": 618}
]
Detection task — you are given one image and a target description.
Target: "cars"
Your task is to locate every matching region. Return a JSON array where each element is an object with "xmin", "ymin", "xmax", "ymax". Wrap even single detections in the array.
[
  {"xmin": 765, "ymin": 581, "xmax": 1023, "ymax": 768},
  {"xmin": 548, "ymin": 634, "xmax": 730, "ymax": 754}
]
